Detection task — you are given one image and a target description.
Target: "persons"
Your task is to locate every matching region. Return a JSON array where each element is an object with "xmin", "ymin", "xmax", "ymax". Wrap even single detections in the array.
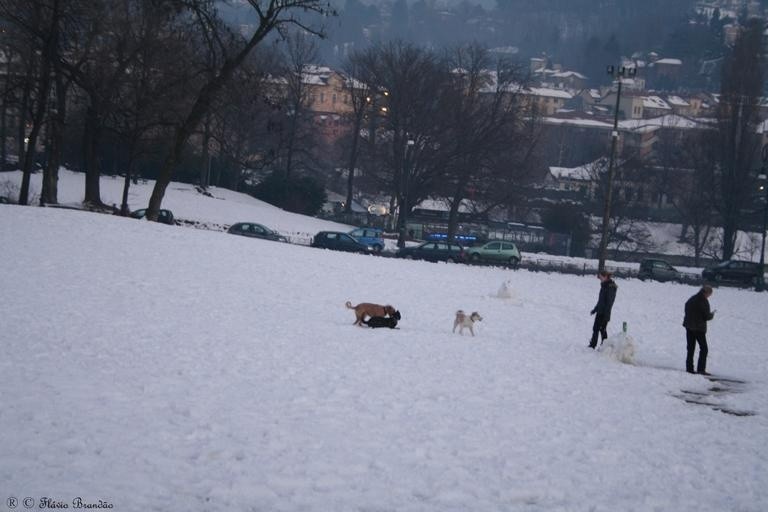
[
  {"xmin": 683, "ymin": 286, "xmax": 717, "ymax": 375},
  {"xmin": 589, "ymin": 270, "xmax": 617, "ymax": 348}
]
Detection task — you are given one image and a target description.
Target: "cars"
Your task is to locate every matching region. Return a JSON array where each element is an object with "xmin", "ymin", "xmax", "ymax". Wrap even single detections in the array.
[
  {"xmin": 227, "ymin": 222, "xmax": 288, "ymax": 243},
  {"xmin": 399, "ymin": 240, "xmax": 466, "ymax": 263},
  {"xmin": 312, "ymin": 231, "xmax": 361, "ymax": 251},
  {"xmin": 637, "ymin": 257, "xmax": 681, "ymax": 282},
  {"xmin": 468, "ymin": 241, "xmax": 521, "ymax": 265},
  {"xmin": 348, "ymin": 227, "xmax": 385, "ymax": 252}
]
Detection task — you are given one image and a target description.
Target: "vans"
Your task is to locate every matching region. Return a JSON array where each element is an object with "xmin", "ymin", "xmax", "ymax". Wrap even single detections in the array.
[{"xmin": 701, "ymin": 259, "xmax": 764, "ymax": 285}]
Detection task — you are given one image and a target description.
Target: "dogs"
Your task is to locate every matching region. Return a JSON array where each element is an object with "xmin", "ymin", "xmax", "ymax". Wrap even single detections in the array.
[
  {"xmin": 453, "ymin": 310, "xmax": 482, "ymax": 336},
  {"xmin": 361, "ymin": 310, "xmax": 401, "ymax": 328},
  {"xmin": 346, "ymin": 301, "xmax": 395, "ymax": 326}
]
